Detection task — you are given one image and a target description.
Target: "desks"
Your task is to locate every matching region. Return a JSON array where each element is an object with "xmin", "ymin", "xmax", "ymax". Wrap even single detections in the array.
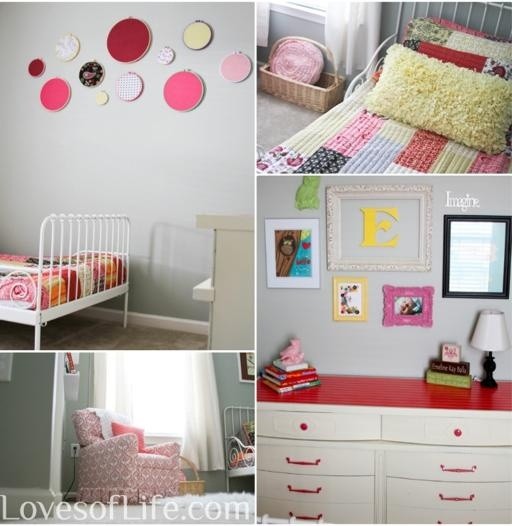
[{"xmin": 192, "ymin": 214, "xmax": 255, "ymax": 349}]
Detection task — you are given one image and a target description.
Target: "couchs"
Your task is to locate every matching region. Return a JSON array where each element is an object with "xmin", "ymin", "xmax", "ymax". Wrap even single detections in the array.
[{"xmin": 70, "ymin": 407, "xmax": 181, "ymax": 505}]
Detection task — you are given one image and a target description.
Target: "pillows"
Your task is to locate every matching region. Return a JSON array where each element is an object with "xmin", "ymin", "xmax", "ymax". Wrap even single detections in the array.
[
  {"xmin": 364, "ymin": 18, "xmax": 512, "ymax": 156},
  {"xmin": 111, "ymin": 422, "xmax": 145, "ymax": 453}
]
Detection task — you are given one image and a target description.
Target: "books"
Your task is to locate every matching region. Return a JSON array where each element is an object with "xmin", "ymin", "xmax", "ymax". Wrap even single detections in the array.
[{"xmin": 261, "ymin": 359, "xmax": 322, "ymax": 394}]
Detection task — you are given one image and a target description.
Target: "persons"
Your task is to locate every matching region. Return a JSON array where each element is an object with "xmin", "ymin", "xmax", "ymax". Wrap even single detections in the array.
[{"xmin": 398, "ymin": 298, "xmax": 418, "ymax": 315}]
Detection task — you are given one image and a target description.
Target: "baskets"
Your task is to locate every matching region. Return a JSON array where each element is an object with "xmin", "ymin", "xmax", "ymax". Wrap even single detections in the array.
[
  {"xmin": 179, "ymin": 456, "xmax": 205, "ymax": 495},
  {"xmin": 259, "ymin": 37, "xmax": 345, "ymax": 113}
]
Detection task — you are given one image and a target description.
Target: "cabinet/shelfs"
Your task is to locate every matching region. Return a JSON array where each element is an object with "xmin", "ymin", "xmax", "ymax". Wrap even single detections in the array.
[
  {"xmin": 257, "ymin": 402, "xmax": 382, "ymax": 524},
  {"xmin": 382, "ymin": 406, "xmax": 512, "ymax": 524}
]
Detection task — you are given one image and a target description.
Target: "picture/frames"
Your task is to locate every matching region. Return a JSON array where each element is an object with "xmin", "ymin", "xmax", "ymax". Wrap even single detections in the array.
[
  {"xmin": 326, "ymin": 183, "xmax": 434, "ymax": 271},
  {"xmin": 333, "ymin": 277, "xmax": 368, "ymax": 322},
  {"xmin": 442, "ymin": 215, "xmax": 512, "ymax": 299},
  {"xmin": 237, "ymin": 352, "xmax": 255, "ymax": 383},
  {"xmin": 382, "ymin": 285, "xmax": 435, "ymax": 328},
  {"xmin": 265, "ymin": 218, "xmax": 320, "ymax": 290}
]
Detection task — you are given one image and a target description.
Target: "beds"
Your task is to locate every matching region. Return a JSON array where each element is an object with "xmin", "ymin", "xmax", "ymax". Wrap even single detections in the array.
[
  {"xmin": 257, "ymin": 2, "xmax": 512, "ymax": 173},
  {"xmin": 223, "ymin": 405, "xmax": 254, "ymax": 492},
  {"xmin": 0, "ymin": 214, "xmax": 131, "ymax": 350}
]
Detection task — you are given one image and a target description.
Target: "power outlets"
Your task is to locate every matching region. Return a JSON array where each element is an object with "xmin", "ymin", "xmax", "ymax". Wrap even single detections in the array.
[{"xmin": 70, "ymin": 444, "xmax": 81, "ymax": 458}]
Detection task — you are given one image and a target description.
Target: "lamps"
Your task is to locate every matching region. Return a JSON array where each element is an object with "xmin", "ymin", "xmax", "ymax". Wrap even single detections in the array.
[{"xmin": 469, "ymin": 309, "xmax": 509, "ymax": 389}]
